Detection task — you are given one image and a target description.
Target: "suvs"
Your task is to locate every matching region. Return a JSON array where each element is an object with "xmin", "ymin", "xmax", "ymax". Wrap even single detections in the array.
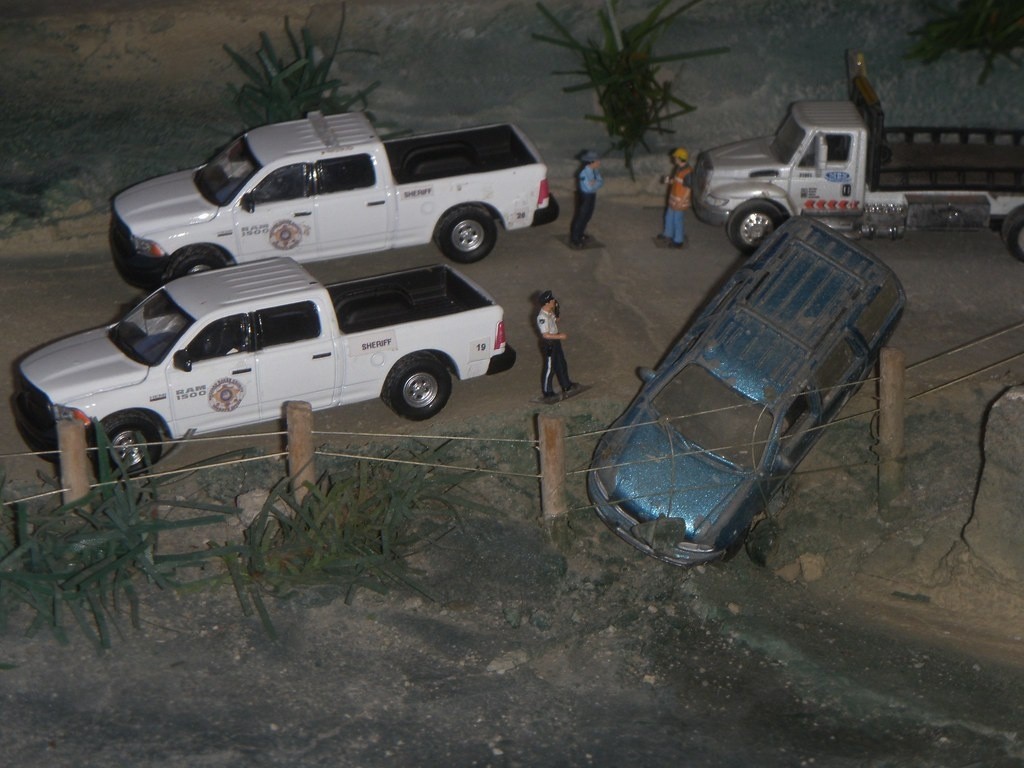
[{"xmin": 586, "ymin": 210, "xmax": 908, "ymax": 570}]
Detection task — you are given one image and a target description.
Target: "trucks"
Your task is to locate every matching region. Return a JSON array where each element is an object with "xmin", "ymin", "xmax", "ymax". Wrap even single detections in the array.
[{"xmin": 693, "ymin": 71, "xmax": 1024, "ymax": 262}]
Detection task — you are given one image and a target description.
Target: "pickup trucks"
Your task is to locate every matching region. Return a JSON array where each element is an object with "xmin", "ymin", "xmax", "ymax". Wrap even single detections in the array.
[
  {"xmin": 107, "ymin": 107, "xmax": 560, "ymax": 291},
  {"xmin": 9, "ymin": 258, "xmax": 516, "ymax": 486}
]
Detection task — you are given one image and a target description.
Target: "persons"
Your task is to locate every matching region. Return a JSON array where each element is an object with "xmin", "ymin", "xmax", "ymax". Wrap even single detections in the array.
[
  {"xmin": 570, "ymin": 150, "xmax": 604, "ymax": 252},
  {"xmin": 657, "ymin": 146, "xmax": 694, "ymax": 250},
  {"xmin": 536, "ymin": 289, "xmax": 578, "ymax": 400}
]
